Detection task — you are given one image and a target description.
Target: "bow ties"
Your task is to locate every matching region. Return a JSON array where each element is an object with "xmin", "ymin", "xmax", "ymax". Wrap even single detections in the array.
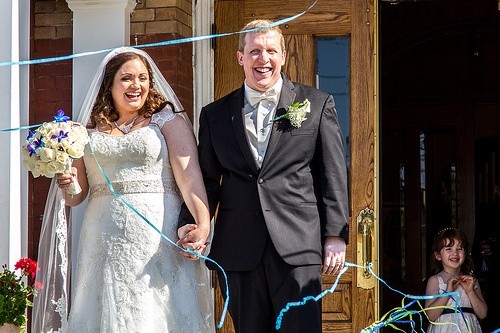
[{"xmin": 247, "ymin": 88, "xmax": 279, "ymax": 108}]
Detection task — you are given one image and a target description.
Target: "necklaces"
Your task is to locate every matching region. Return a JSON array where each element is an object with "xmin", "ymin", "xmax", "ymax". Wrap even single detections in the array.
[{"xmin": 114, "ymin": 115, "xmax": 139, "ymax": 134}]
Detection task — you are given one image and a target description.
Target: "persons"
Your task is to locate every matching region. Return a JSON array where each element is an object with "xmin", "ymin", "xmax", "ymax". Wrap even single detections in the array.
[
  {"xmin": 32, "ymin": 48, "xmax": 216, "ymax": 333},
  {"xmin": 177, "ymin": 20, "xmax": 349, "ymax": 333},
  {"xmin": 425, "ymin": 226, "xmax": 500, "ymax": 333}
]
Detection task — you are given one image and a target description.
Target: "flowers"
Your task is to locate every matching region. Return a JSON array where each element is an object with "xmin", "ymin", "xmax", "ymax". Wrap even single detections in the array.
[
  {"xmin": 270, "ymin": 96, "xmax": 311, "ymax": 128},
  {"xmin": 22, "ymin": 108, "xmax": 91, "ymax": 196}
]
